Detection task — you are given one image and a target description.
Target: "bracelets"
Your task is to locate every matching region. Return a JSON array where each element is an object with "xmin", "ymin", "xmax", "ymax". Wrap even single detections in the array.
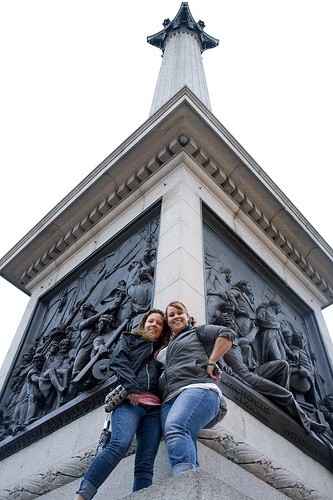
[{"xmin": 207, "ymin": 362, "xmax": 215, "ymax": 366}]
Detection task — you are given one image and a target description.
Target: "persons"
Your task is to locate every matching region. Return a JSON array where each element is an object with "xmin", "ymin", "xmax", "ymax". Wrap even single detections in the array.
[
  {"xmin": 74, "ymin": 308, "xmax": 173, "ymax": 500},
  {"xmin": 153, "ymin": 301, "xmax": 237, "ymax": 476},
  {"xmin": 6, "ymin": 257, "xmax": 155, "ymax": 436},
  {"xmin": 209, "ymin": 280, "xmax": 333, "ymax": 443}
]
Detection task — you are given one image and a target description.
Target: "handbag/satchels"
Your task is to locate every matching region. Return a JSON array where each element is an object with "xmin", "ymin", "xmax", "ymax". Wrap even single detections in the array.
[{"xmin": 104, "ymin": 338, "xmax": 162, "ymax": 413}]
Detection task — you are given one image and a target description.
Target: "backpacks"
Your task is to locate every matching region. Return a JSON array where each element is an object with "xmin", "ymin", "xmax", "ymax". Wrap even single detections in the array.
[{"xmin": 190, "ymin": 325, "xmax": 227, "ymax": 429}]
